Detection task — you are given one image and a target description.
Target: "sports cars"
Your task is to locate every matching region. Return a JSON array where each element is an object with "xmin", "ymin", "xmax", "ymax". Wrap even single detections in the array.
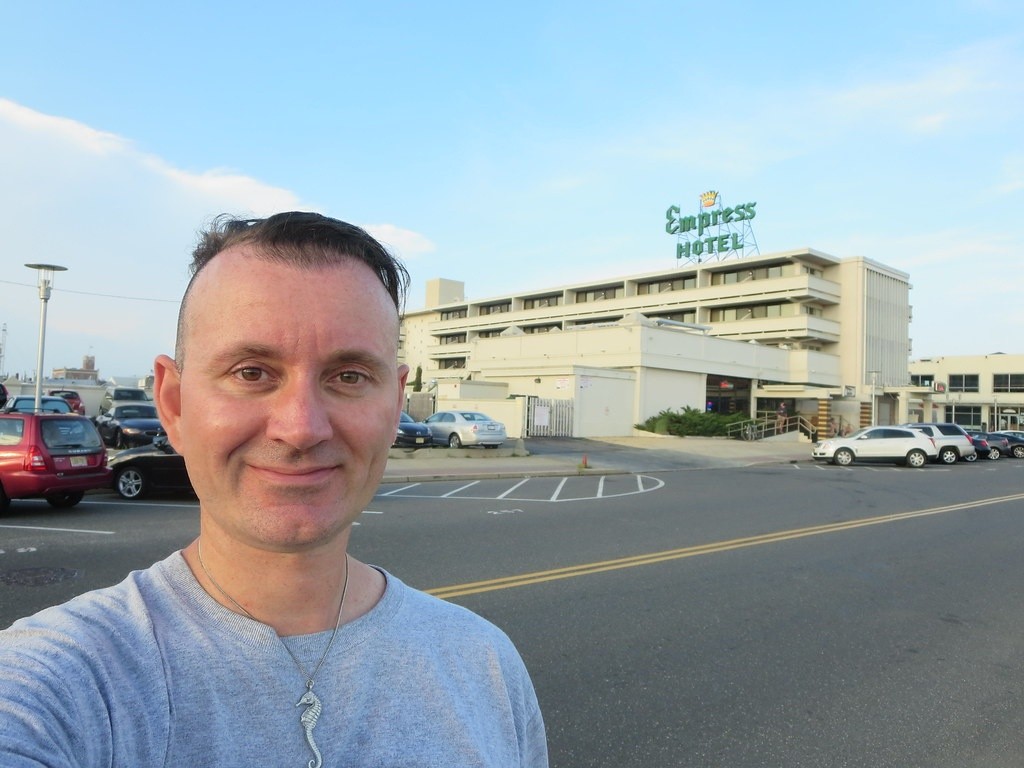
[{"xmin": 394, "ymin": 412, "xmax": 434, "ymax": 448}]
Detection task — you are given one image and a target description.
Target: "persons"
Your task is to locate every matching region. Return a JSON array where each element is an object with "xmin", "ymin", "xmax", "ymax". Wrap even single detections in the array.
[{"xmin": 0, "ymin": 212, "xmax": 551, "ymax": 768}]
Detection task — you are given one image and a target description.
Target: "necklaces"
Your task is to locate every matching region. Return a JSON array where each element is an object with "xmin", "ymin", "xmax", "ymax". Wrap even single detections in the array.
[{"xmin": 197, "ymin": 540, "xmax": 349, "ymax": 768}]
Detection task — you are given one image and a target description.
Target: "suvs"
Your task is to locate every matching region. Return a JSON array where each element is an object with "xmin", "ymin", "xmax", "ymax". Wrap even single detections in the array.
[
  {"xmin": 99, "ymin": 387, "xmax": 153, "ymax": 415},
  {"xmin": 0, "ymin": 409, "xmax": 114, "ymax": 515},
  {"xmin": 0, "ymin": 396, "xmax": 86, "ymax": 441},
  {"xmin": 898, "ymin": 423, "xmax": 976, "ymax": 467},
  {"xmin": 811, "ymin": 426, "xmax": 938, "ymax": 468}
]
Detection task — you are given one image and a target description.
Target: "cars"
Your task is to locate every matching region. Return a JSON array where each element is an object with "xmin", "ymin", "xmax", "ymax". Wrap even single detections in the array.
[
  {"xmin": 958, "ymin": 437, "xmax": 992, "ymax": 463},
  {"xmin": 966, "ymin": 431, "xmax": 1012, "ymax": 461},
  {"xmin": 44, "ymin": 389, "xmax": 85, "ymax": 415},
  {"xmin": 989, "ymin": 432, "xmax": 1024, "ymax": 458},
  {"xmin": 106, "ymin": 437, "xmax": 200, "ymax": 500},
  {"xmin": 997, "ymin": 431, "xmax": 1024, "ymax": 438},
  {"xmin": 420, "ymin": 411, "xmax": 506, "ymax": 450},
  {"xmin": 94, "ymin": 403, "xmax": 169, "ymax": 449}
]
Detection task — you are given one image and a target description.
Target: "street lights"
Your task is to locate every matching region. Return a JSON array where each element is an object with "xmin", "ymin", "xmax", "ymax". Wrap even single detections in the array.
[{"xmin": 24, "ymin": 263, "xmax": 68, "ymax": 416}]
